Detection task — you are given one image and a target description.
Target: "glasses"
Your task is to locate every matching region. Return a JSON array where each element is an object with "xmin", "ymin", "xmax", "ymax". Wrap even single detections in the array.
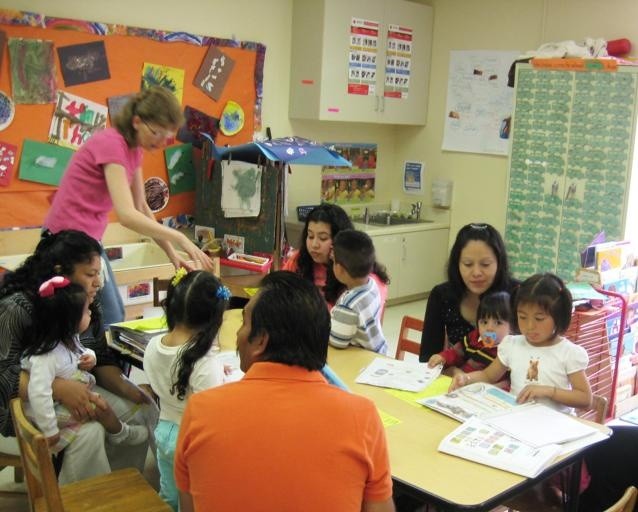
[{"xmin": 140, "ymin": 116, "xmax": 161, "ymax": 138}]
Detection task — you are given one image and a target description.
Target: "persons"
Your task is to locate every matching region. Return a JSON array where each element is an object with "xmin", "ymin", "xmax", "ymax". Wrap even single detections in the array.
[
  {"xmin": 321, "ymin": 179, "xmax": 376, "ymax": 203},
  {"xmin": 328, "ymin": 230, "xmax": 387, "ymax": 357},
  {"xmin": 35, "ymin": 85, "xmax": 213, "ymax": 374},
  {"xmin": 427, "ymin": 291, "xmax": 523, "ymax": 393},
  {"xmin": 21, "ymin": 278, "xmax": 150, "ymax": 449},
  {"xmin": 0, "ymin": 228, "xmax": 162, "ymax": 496},
  {"xmin": 283, "ymin": 202, "xmax": 386, "ymax": 331},
  {"xmin": 447, "ymin": 271, "xmax": 592, "ymax": 511},
  {"xmin": 173, "ymin": 269, "xmax": 398, "ymax": 511},
  {"xmin": 142, "ymin": 272, "xmax": 232, "ymax": 511},
  {"xmin": 419, "ymin": 223, "xmax": 522, "ymax": 364}
]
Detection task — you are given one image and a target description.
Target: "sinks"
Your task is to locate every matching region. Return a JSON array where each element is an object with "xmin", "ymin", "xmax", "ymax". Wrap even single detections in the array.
[{"xmin": 353, "ymin": 217, "xmax": 434, "ymax": 226}]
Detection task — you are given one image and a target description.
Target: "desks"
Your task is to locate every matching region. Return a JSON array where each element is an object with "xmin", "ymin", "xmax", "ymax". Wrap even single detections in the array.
[{"xmin": 214, "ymin": 308, "xmax": 614, "ymax": 512}]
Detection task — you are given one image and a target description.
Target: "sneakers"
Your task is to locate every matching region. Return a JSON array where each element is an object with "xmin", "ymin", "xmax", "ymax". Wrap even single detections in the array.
[{"xmin": 108, "ymin": 422, "xmax": 149, "ymax": 447}]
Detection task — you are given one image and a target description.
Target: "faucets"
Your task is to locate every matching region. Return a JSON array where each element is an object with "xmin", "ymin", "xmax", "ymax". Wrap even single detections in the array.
[{"xmin": 411, "ymin": 201, "xmax": 422, "ymax": 221}]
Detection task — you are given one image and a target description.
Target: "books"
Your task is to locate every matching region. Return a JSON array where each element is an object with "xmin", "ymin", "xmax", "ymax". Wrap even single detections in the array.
[
  {"xmin": 417, "ymin": 380, "xmax": 611, "ymax": 479},
  {"xmin": 106, "ymin": 314, "xmax": 172, "ymax": 362},
  {"xmin": 353, "ymin": 354, "xmax": 446, "ymax": 394},
  {"xmin": 577, "ymin": 229, "xmax": 638, "ymax": 403}
]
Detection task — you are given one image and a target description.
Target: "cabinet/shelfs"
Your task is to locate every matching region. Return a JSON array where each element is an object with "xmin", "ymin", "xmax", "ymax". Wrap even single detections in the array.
[
  {"xmin": 504, "ymin": 62, "xmax": 638, "ymax": 281},
  {"xmin": 285, "ymin": 211, "xmax": 450, "ymax": 307},
  {"xmin": 562, "ymin": 292, "xmax": 638, "ymax": 425},
  {"xmin": 289, "ymin": 0, "xmax": 434, "ymax": 125}
]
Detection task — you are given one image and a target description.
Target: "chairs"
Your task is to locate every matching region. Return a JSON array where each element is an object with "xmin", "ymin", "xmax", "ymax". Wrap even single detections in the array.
[
  {"xmin": 580, "ymin": 393, "xmax": 609, "ymax": 424},
  {"xmin": 153, "ymin": 277, "xmax": 172, "ymax": 307},
  {"xmin": 0, "ymin": 456, "xmax": 27, "ymax": 498},
  {"xmin": 395, "ymin": 315, "xmax": 424, "ymax": 360},
  {"xmin": 9, "ymin": 396, "xmax": 173, "ymax": 512}
]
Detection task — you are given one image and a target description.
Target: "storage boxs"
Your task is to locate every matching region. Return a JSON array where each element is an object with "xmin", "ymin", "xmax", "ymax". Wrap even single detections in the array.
[{"xmin": 220, "ymin": 251, "xmax": 274, "ymax": 277}]
